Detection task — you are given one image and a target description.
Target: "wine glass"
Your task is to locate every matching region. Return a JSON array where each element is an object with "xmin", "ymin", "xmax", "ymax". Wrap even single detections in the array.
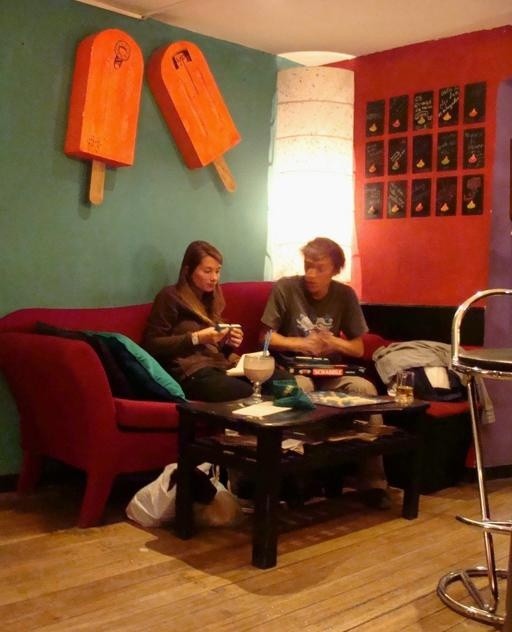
[{"xmin": 243, "ymin": 354, "xmax": 274, "ymax": 407}]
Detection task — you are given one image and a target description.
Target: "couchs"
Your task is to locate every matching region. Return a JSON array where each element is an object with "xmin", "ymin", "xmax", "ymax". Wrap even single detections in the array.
[{"xmin": 0, "ymin": 280, "xmax": 486, "ymax": 530}]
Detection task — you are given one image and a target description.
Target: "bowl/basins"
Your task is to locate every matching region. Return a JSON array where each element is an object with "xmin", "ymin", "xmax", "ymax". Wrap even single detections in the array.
[{"xmin": 273, "ymin": 380, "xmax": 298, "ymax": 400}]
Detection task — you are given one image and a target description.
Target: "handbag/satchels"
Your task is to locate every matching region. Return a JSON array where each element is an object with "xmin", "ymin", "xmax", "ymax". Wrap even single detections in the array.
[
  {"xmin": 192, "ymin": 475, "xmax": 248, "ymax": 529},
  {"xmin": 127, "ymin": 459, "xmax": 211, "ymax": 528}
]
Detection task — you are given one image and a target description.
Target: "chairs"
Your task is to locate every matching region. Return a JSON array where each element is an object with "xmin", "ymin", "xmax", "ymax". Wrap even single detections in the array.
[{"xmin": 436, "ymin": 288, "xmax": 512, "ymax": 626}]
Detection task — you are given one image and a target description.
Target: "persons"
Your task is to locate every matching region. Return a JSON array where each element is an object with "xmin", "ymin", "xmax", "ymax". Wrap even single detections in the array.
[
  {"xmin": 140, "ymin": 240, "xmax": 297, "ymax": 403},
  {"xmin": 260, "ymin": 238, "xmax": 392, "ymax": 511}
]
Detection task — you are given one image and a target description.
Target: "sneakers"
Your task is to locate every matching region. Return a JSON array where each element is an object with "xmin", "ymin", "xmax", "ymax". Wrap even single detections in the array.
[{"xmin": 359, "ymin": 486, "xmax": 390, "ymax": 510}]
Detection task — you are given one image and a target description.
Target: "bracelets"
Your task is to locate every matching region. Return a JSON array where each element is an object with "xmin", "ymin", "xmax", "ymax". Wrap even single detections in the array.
[{"xmin": 191, "ymin": 330, "xmax": 200, "ymax": 346}]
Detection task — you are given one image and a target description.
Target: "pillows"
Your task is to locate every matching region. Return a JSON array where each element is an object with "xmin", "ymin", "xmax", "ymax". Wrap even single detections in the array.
[{"xmin": 33, "ymin": 320, "xmax": 190, "ymax": 403}]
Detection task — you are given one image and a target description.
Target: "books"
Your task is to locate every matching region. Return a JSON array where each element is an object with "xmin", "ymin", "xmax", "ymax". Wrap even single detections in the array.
[{"xmin": 278, "ymin": 355, "xmax": 365, "ymax": 378}]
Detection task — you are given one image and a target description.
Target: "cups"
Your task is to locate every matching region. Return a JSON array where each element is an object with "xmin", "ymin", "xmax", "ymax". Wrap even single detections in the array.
[{"xmin": 396, "ymin": 370, "xmax": 414, "ymax": 404}]
